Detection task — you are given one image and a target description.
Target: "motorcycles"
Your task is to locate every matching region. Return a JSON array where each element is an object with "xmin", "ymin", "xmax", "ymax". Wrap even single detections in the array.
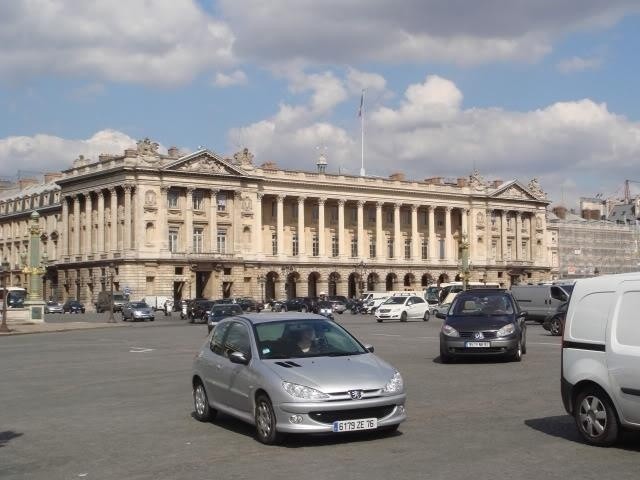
[
  {"xmin": 163, "ymin": 303, "xmax": 172, "ymax": 316},
  {"xmin": 180, "ymin": 302, "xmax": 188, "ymax": 319}
]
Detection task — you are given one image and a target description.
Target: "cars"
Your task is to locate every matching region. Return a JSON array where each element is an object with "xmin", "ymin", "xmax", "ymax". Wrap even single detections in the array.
[
  {"xmin": 63, "ymin": 300, "xmax": 86, "ymax": 314},
  {"xmin": 122, "ymin": 302, "xmax": 155, "ymax": 322},
  {"xmin": 191, "ymin": 311, "xmax": 407, "ymax": 445},
  {"xmin": 45, "ymin": 301, "xmax": 63, "ymax": 314},
  {"xmin": 437, "ymin": 289, "xmax": 529, "ymax": 364}
]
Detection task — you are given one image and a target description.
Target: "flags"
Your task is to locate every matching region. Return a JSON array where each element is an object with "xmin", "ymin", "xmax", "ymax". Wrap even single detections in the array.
[{"xmin": 358, "ymin": 93, "xmax": 363, "ymax": 116}]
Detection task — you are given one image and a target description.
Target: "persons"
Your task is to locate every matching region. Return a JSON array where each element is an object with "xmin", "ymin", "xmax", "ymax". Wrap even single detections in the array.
[
  {"xmin": 291, "ymin": 324, "xmax": 324, "ymax": 357},
  {"xmin": 317, "ymin": 292, "xmax": 327, "ymax": 303}
]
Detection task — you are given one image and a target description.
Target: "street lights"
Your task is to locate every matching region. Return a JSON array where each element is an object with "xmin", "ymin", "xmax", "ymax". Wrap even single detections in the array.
[
  {"xmin": 256, "ymin": 274, "xmax": 268, "ymax": 305},
  {"xmin": 354, "ymin": 260, "xmax": 367, "ymax": 297},
  {"xmin": 593, "ymin": 266, "xmax": 600, "ymax": 277},
  {"xmin": 464, "ymin": 268, "xmax": 471, "ymax": 290},
  {"xmin": 481, "ymin": 271, "xmax": 488, "ymax": 289},
  {"xmin": 105, "ymin": 261, "xmax": 117, "ymax": 323},
  {"xmin": 1, "ymin": 256, "xmax": 12, "ymax": 332}
]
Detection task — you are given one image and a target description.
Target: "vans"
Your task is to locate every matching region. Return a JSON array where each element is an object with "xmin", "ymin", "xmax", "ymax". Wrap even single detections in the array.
[{"xmin": 561, "ymin": 270, "xmax": 639, "ymax": 446}]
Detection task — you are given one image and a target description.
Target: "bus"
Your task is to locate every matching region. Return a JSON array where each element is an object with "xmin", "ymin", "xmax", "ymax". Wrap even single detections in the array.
[{"xmin": 0, "ymin": 287, "xmax": 30, "ymax": 314}]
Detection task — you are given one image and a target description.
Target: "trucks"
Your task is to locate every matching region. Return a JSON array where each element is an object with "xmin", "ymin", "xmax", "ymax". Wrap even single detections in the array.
[
  {"xmin": 96, "ymin": 290, "xmax": 128, "ymax": 313},
  {"xmin": 509, "ymin": 283, "xmax": 572, "ymax": 336}
]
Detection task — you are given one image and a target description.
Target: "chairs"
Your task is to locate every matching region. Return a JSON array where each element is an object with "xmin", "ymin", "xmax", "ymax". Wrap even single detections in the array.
[{"xmin": 462, "ymin": 301, "xmax": 478, "ymax": 314}]
